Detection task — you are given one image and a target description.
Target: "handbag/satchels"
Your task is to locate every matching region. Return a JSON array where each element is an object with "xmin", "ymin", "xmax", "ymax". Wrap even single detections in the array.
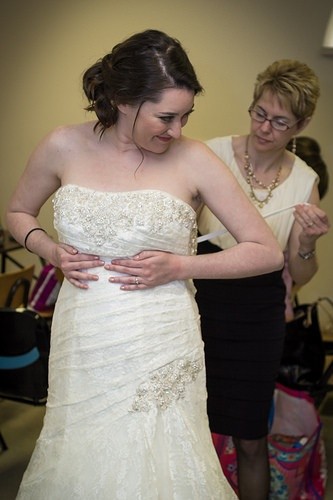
[
  {"xmin": 29, "ymin": 264, "xmax": 65, "ymax": 312},
  {"xmin": 210, "ymin": 385, "xmax": 328, "ymax": 500},
  {"xmin": 282, "ymin": 288, "xmax": 333, "ymax": 394},
  {"xmin": 0, "ymin": 277, "xmax": 52, "ymax": 405}
]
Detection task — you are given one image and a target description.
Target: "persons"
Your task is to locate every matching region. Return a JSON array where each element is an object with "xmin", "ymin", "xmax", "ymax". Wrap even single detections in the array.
[
  {"xmin": 1, "ymin": 30, "xmax": 283, "ymax": 500},
  {"xmin": 194, "ymin": 61, "xmax": 329, "ymax": 500}
]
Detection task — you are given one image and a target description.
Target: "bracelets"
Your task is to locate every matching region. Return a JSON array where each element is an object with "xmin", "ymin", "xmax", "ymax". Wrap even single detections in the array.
[
  {"xmin": 295, "ymin": 246, "xmax": 317, "ymax": 263},
  {"xmin": 24, "ymin": 227, "xmax": 46, "ymax": 252}
]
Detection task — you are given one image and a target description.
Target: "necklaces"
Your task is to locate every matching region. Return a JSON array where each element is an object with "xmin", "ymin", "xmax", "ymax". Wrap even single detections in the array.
[{"xmin": 245, "ymin": 135, "xmax": 284, "ymax": 209}]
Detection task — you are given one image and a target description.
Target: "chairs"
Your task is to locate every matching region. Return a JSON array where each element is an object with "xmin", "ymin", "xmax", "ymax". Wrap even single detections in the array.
[{"xmin": 0, "ymin": 230, "xmax": 54, "ymax": 317}]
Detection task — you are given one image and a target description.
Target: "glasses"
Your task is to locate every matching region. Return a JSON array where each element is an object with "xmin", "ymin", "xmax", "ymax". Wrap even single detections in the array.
[{"xmin": 248, "ymin": 106, "xmax": 304, "ymax": 132}]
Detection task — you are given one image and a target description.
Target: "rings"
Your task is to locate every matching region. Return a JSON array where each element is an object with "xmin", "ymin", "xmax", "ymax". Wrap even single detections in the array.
[{"xmin": 136, "ymin": 277, "xmax": 140, "ymax": 285}]
